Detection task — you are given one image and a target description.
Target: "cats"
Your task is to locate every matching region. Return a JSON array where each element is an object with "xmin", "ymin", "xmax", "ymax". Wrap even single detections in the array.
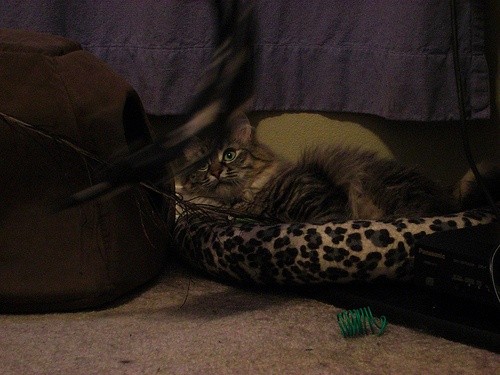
[{"xmin": 170, "ymin": 108, "xmax": 427, "ymax": 223}]
